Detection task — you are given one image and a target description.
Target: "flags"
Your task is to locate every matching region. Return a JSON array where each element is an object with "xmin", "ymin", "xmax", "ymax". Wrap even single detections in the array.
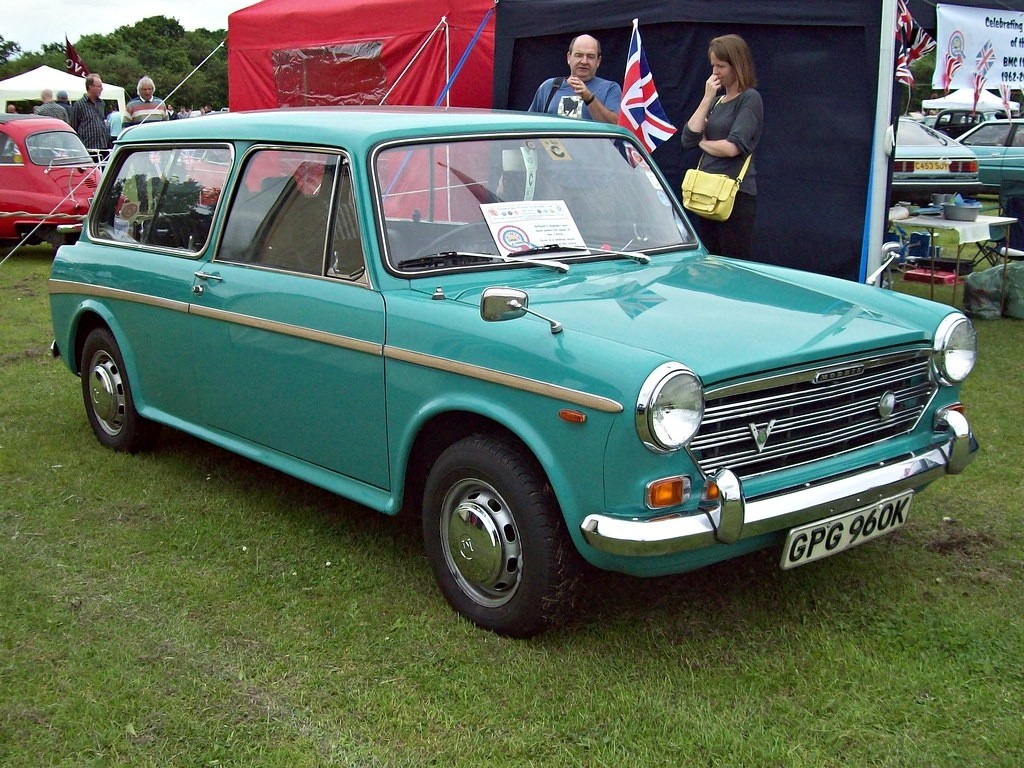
[
  {"xmin": 971, "ymin": 71, "xmax": 989, "ymax": 115},
  {"xmin": 997, "ymin": 82, "xmax": 1013, "ymax": 128},
  {"xmin": 608, "ymin": 19, "xmax": 678, "ymax": 170},
  {"xmin": 942, "ymin": 52, "xmax": 964, "ymax": 99},
  {"xmin": 894, "ymin": 0, "xmax": 938, "ymax": 91},
  {"xmin": 974, "ymin": 37, "xmax": 996, "ymax": 77}
]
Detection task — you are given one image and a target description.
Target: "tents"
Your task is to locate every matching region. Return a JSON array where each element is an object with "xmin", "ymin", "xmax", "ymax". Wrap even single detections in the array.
[
  {"xmin": 0, "ymin": 64, "xmax": 126, "ymax": 118},
  {"xmin": 495, "ymin": 0, "xmax": 934, "ymax": 289},
  {"xmin": 922, "ymin": 87, "xmax": 1020, "ymax": 118},
  {"xmin": 227, "ymin": 0, "xmax": 493, "ymax": 230}
]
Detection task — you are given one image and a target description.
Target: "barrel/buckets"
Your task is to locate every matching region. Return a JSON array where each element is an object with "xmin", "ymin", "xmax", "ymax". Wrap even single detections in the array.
[{"xmin": 908, "ymin": 232, "xmax": 940, "ymax": 257}]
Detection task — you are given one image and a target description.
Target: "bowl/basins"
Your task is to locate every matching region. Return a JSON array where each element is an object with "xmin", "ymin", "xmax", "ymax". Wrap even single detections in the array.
[{"xmin": 940, "ymin": 202, "xmax": 982, "ymax": 221}]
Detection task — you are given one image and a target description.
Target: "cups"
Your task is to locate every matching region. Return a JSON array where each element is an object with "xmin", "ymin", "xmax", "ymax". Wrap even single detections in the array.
[
  {"xmin": 945, "ymin": 194, "xmax": 954, "ymax": 201},
  {"xmin": 930, "ymin": 193, "xmax": 946, "ymax": 205},
  {"xmin": 895, "ymin": 202, "xmax": 911, "ymax": 215}
]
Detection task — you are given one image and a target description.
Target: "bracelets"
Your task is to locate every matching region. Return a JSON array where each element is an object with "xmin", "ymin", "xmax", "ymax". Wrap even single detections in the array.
[{"xmin": 584, "ymin": 93, "xmax": 594, "ymax": 105}]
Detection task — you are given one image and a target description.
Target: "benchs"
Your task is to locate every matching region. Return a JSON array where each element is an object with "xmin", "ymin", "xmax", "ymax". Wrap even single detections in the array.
[{"xmin": 133, "ymin": 209, "xmax": 212, "ymax": 252}]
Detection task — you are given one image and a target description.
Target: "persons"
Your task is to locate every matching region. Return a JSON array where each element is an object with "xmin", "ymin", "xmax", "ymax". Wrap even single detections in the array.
[
  {"xmin": 527, "ymin": 33, "xmax": 622, "ymax": 124},
  {"xmin": 107, "ymin": 105, "xmax": 123, "ymax": 137},
  {"xmin": 68, "ymin": 73, "xmax": 106, "ymax": 162},
  {"xmin": 122, "ymin": 76, "xmax": 170, "ymax": 216},
  {"xmin": 166, "ymin": 104, "xmax": 212, "ymax": 120},
  {"xmin": 8, "ymin": 105, "xmax": 19, "ymax": 113},
  {"xmin": 35, "ymin": 90, "xmax": 73, "ymax": 125},
  {"xmin": 677, "ymin": 33, "xmax": 764, "ymax": 259}
]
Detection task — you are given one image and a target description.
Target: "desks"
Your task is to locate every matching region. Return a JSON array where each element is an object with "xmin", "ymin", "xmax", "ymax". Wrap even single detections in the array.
[{"xmin": 895, "ymin": 215, "xmax": 1017, "ymax": 317}]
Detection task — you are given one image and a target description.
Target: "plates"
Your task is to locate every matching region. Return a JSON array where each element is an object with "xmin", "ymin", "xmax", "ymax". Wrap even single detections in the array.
[
  {"xmin": 915, "ymin": 208, "xmax": 940, "ymax": 214},
  {"xmin": 928, "ymin": 202, "xmax": 945, "ymax": 209}
]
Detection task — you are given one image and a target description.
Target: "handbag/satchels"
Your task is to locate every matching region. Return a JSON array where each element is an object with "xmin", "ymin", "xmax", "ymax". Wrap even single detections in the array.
[{"xmin": 681, "ymin": 169, "xmax": 740, "ymax": 221}]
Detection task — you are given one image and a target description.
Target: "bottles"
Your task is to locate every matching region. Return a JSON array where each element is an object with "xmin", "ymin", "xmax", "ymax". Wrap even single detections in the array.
[{"xmin": 910, "ymin": 204, "xmax": 921, "ymax": 215}]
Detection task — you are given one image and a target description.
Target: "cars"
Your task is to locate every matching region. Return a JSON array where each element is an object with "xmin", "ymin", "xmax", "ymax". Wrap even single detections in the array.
[
  {"xmin": 47, "ymin": 103, "xmax": 977, "ymax": 639},
  {"xmin": 0, "ymin": 110, "xmax": 131, "ymax": 244},
  {"xmin": 889, "ymin": 107, "xmax": 1024, "ymax": 204}
]
